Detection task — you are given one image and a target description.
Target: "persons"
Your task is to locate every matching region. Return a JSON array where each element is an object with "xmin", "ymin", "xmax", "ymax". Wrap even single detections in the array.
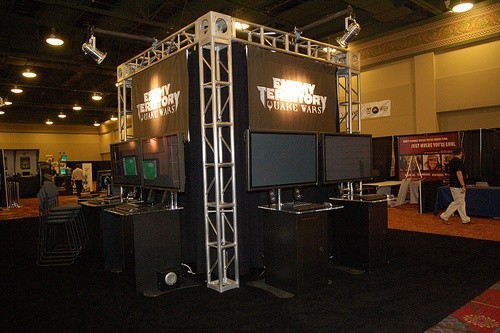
[
  {"xmin": 440, "ymin": 146, "xmax": 475, "ymax": 225},
  {"xmin": 71, "ymin": 164, "xmax": 86, "ymax": 199},
  {"xmin": 426, "ymin": 155, "xmax": 441, "ymax": 170}
]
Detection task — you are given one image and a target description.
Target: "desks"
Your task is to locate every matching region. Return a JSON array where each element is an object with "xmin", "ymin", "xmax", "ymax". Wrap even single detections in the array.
[
  {"xmin": 362, "ymin": 180, "xmax": 402, "ymax": 194},
  {"xmin": 104, "ymin": 202, "xmax": 184, "ymax": 293},
  {"xmin": 82, "ymin": 205, "xmax": 122, "ymax": 270},
  {"xmin": 329, "ymin": 197, "xmax": 389, "ymax": 273},
  {"xmin": 258, "ymin": 202, "xmax": 344, "ymax": 298},
  {"xmin": 434, "ymin": 184, "xmax": 500, "ymax": 219}
]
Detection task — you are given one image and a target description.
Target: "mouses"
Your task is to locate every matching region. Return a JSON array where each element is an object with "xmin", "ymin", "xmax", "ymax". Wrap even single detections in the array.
[
  {"xmin": 101, "ymin": 201, "xmax": 107, "ymax": 204},
  {"xmin": 322, "ymin": 202, "xmax": 333, "ymax": 208},
  {"xmin": 129, "ymin": 209, "xmax": 136, "ymax": 212}
]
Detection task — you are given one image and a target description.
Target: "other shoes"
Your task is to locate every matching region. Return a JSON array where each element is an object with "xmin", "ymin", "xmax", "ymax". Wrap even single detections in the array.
[
  {"xmin": 462, "ymin": 220, "xmax": 471, "ymax": 224},
  {"xmin": 440, "ymin": 217, "xmax": 451, "ymax": 225}
]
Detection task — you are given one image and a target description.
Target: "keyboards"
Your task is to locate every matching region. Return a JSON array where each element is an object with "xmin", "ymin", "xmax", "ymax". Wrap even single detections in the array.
[
  {"xmin": 115, "ymin": 202, "xmax": 138, "ymax": 211},
  {"xmin": 289, "ymin": 203, "xmax": 328, "ymax": 212},
  {"xmin": 88, "ymin": 200, "xmax": 109, "ymax": 205},
  {"xmin": 357, "ymin": 193, "xmax": 391, "ymax": 202}
]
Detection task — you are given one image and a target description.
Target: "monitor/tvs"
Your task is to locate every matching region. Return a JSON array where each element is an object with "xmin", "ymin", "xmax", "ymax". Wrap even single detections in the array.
[
  {"xmin": 109, "ymin": 138, "xmax": 143, "ymax": 199},
  {"xmin": 322, "ymin": 133, "xmax": 374, "ymax": 199},
  {"xmin": 140, "ymin": 130, "xmax": 184, "ymax": 206},
  {"xmin": 245, "ymin": 128, "xmax": 319, "ymax": 207}
]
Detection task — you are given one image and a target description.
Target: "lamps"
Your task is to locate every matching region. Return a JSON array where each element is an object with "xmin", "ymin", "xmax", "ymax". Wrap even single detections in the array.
[
  {"xmin": 93, "ymin": 121, "xmax": 101, "ymax": 127},
  {"xmin": 21, "ymin": 65, "xmax": 38, "ymax": 79},
  {"xmin": 10, "ymin": 86, "xmax": 24, "ymax": 94},
  {"xmin": 92, "ymin": 93, "xmax": 102, "ymax": 101},
  {"xmin": 110, "ymin": 115, "xmax": 118, "ymax": 122},
  {"xmin": 45, "ymin": 119, "xmax": 54, "ymax": 125},
  {"xmin": 45, "ymin": 25, "xmax": 65, "ymax": 47},
  {"xmin": 81, "ymin": 26, "xmax": 108, "ymax": 65},
  {"xmin": 4, "ymin": 98, "xmax": 12, "ymax": 106},
  {"xmin": 73, "ymin": 104, "xmax": 82, "ymax": 111},
  {"xmin": 57, "ymin": 112, "xmax": 67, "ymax": 119},
  {"xmin": 336, "ymin": 13, "xmax": 362, "ymax": 50},
  {"xmin": 450, "ymin": 0, "xmax": 474, "ymax": 13}
]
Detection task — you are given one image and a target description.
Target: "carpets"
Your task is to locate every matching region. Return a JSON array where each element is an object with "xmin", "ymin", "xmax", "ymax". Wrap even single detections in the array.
[{"xmin": 0, "ymin": 215, "xmax": 500, "ymax": 333}]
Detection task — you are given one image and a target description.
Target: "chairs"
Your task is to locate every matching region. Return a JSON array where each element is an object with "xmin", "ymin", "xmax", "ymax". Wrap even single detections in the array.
[{"xmin": 36, "ymin": 181, "xmax": 91, "ymax": 267}]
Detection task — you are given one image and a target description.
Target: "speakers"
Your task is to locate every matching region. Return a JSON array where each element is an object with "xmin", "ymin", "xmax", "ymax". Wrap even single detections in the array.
[{"xmin": 157, "ymin": 268, "xmax": 180, "ymax": 291}]
